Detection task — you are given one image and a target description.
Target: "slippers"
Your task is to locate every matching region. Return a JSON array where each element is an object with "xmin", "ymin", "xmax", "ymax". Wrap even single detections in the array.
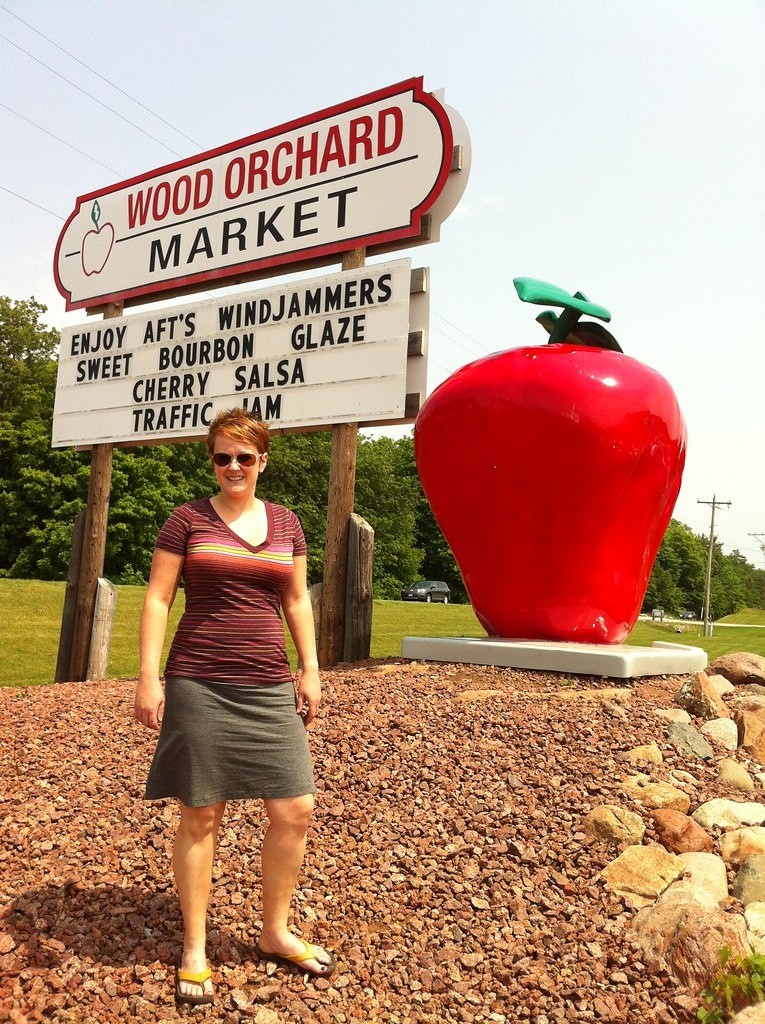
[
  {"xmin": 177, "ymin": 966, "xmax": 216, "ymax": 1002},
  {"xmin": 255, "ymin": 938, "xmax": 336, "ymax": 976}
]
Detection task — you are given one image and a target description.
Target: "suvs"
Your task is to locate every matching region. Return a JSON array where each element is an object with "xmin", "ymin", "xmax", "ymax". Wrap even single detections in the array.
[{"xmin": 401, "ymin": 581, "xmax": 451, "ymax": 604}]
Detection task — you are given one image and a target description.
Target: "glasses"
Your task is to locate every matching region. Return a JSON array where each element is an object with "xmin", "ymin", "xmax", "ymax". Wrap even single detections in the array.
[{"xmin": 211, "ymin": 453, "xmax": 262, "ymax": 467}]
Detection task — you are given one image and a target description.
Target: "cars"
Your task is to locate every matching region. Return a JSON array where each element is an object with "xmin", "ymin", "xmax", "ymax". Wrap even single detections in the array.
[{"xmin": 680, "ymin": 611, "xmax": 696, "ymax": 621}]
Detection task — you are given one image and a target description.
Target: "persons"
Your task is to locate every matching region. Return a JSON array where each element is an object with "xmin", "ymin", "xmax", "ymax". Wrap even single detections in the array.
[{"xmin": 134, "ymin": 407, "xmax": 336, "ymax": 1004}]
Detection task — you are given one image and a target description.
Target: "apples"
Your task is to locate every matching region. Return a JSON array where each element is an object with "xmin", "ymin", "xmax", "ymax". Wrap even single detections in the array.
[{"xmin": 412, "ymin": 277, "xmax": 688, "ymax": 645}]
